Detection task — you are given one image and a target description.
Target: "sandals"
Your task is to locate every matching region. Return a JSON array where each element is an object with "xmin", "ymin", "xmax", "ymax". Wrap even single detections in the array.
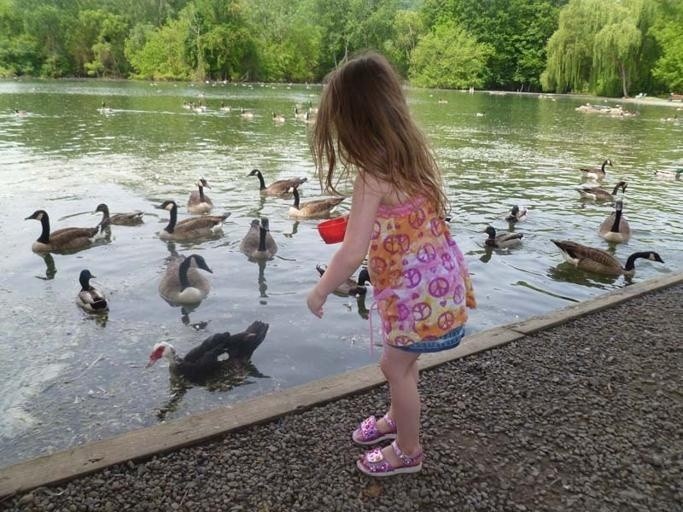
[
  {"xmin": 356, "ymin": 440, "xmax": 423, "ymax": 476},
  {"xmin": 352, "ymin": 413, "xmax": 396, "ymax": 445}
]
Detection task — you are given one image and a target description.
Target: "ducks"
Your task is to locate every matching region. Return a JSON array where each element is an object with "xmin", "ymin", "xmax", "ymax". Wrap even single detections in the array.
[
  {"xmin": 505, "ymin": 205, "xmax": 527, "ymax": 222},
  {"xmin": 101, "ymin": 101, "xmax": 113, "ymax": 114},
  {"xmin": 478, "ymin": 225, "xmax": 526, "ymax": 248},
  {"xmin": 14, "ymin": 108, "xmax": 28, "ymax": 117}
]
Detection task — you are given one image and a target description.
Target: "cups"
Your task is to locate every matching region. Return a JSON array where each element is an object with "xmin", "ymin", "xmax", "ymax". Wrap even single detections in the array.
[{"xmin": 317, "ymin": 217, "xmax": 347, "ymax": 244}]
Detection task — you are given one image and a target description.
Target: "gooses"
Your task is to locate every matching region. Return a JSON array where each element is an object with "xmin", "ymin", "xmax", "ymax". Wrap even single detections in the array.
[
  {"xmin": 91, "ymin": 201, "xmax": 144, "ymax": 227},
  {"xmin": 281, "ymin": 184, "xmax": 345, "ymax": 220},
  {"xmin": 74, "ymin": 268, "xmax": 111, "ymax": 314},
  {"xmin": 313, "ymin": 263, "xmax": 373, "ymax": 297},
  {"xmin": 549, "ymin": 159, "xmax": 669, "ymax": 279},
  {"xmin": 157, "ymin": 250, "xmax": 213, "ymax": 307},
  {"xmin": 246, "ymin": 168, "xmax": 308, "ymax": 197},
  {"xmin": 154, "ymin": 199, "xmax": 232, "ymax": 241},
  {"xmin": 178, "ymin": 97, "xmax": 318, "ymax": 123},
  {"xmin": 24, "ymin": 209, "xmax": 110, "ymax": 252},
  {"xmin": 240, "ymin": 216, "xmax": 277, "ymax": 261},
  {"xmin": 144, "ymin": 319, "xmax": 270, "ymax": 383},
  {"xmin": 186, "ymin": 177, "xmax": 214, "ymax": 214}
]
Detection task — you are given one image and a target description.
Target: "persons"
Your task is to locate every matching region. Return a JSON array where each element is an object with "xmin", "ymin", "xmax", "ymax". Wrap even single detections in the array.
[{"xmin": 304, "ymin": 46, "xmax": 470, "ymax": 477}]
